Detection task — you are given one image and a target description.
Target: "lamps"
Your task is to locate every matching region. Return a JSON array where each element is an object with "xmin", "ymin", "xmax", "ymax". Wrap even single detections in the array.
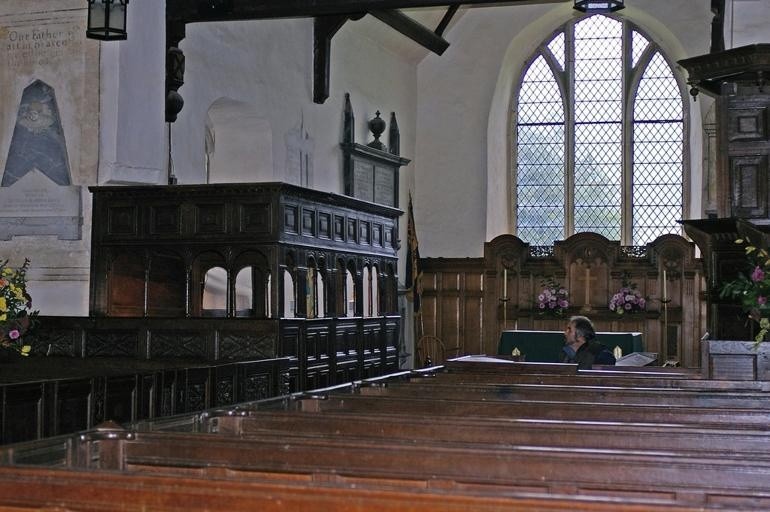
[{"xmin": 85, "ymin": 0, "xmax": 129, "ymax": 43}]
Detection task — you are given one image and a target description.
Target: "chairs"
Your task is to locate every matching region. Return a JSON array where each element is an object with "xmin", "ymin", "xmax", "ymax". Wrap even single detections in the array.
[{"xmin": 417, "ymin": 335, "xmax": 461, "ymax": 368}]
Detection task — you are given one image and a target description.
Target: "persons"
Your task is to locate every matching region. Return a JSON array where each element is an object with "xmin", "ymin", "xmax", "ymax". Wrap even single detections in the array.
[{"xmin": 561, "ymin": 314, "xmax": 618, "ymax": 365}]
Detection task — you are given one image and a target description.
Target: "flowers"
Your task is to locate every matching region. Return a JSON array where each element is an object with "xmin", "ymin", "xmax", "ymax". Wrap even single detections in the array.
[
  {"xmin": 608, "ymin": 269, "xmax": 646, "ymax": 315},
  {"xmin": 717, "ymin": 235, "xmax": 769, "ymax": 350},
  {"xmin": 0, "ymin": 257, "xmax": 41, "ymax": 358},
  {"xmin": 535, "ymin": 275, "xmax": 571, "ymax": 319}
]
